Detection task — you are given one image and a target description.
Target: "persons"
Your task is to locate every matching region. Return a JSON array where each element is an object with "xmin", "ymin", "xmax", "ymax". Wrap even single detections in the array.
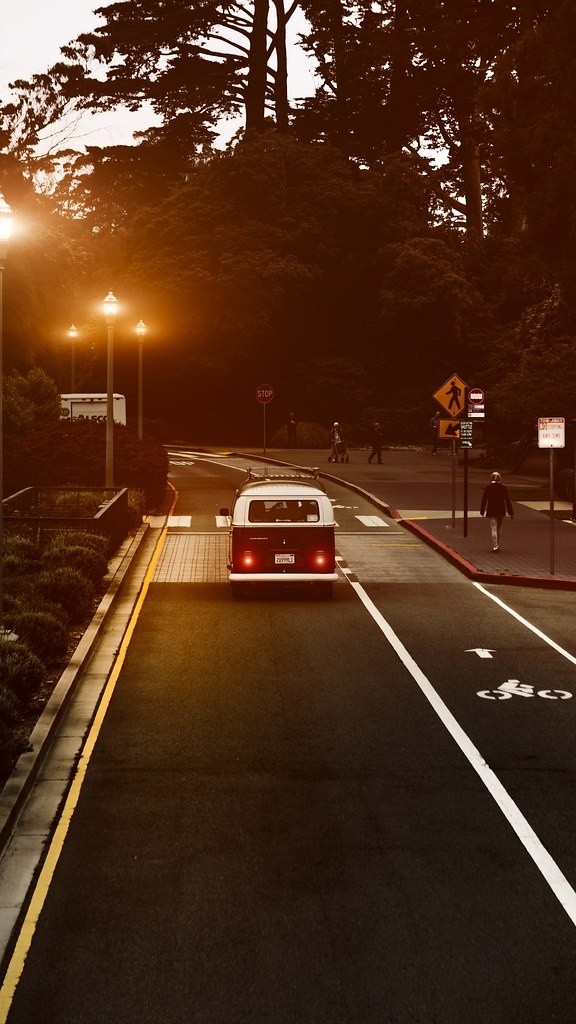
[
  {"xmin": 327, "ymin": 422, "xmax": 343, "ymax": 462},
  {"xmin": 368, "ymin": 423, "xmax": 383, "ymax": 464},
  {"xmin": 287, "ymin": 412, "xmax": 297, "ymax": 444},
  {"xmin": 448, "ymin": 440, "xmax": 458, "ymax": 456},
  {"xmin": 428, "ymin": 412, "xmax": 441, "ymax": 455},
  {"xmin": 480, "ymin": 472, "xmax": 515, "ymax": 552}
]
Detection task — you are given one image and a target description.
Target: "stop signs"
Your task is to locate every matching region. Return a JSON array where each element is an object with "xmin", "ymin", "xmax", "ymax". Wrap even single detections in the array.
[
  {"xmin": 257, "ymin": 383, "xmax": 275, "ymax": 403},
  {"xmin": 467, "ymin": 388, "xmax": 484, "ymax": 404}
]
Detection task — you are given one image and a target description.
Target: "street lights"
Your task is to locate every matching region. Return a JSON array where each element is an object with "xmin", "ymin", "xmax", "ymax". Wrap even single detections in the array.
[
  {"xmin": 134, "ymin": 319, "xmax": 147, "ymax": 442},
  {"xmin": 101, "ymin": 288, "xmax": 118, "ymax": 500},
  {"xmin": 67, "ymin": 323, "xmax": 79, "ymax": 396}
]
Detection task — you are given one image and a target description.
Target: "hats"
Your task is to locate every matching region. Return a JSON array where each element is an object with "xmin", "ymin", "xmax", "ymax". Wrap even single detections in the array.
[{"xmin": 334, "ymin": 422, "xmax": 339, "ymax": 427}]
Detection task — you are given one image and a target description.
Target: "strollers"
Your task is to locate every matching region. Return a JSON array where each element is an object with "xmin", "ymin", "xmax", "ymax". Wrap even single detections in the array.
[{"xmin": 332, "ymin": 437, "xmax": 351, "ymax": 463}]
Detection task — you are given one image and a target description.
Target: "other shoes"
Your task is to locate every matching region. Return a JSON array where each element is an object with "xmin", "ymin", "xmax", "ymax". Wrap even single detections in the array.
[
  {"xmin": 368, "ymin": 457, "xmax": 371, "ymax": 463},
  {"xmin": 455, "ymin": 453, "xmax": 457, "ymax": 456},
  {"xmin": 333, "ymin": 458, "xmax": 336, "ymax": 462},
  {"xmin": 377, "ymin": 463, "xmax": 383, "ymax": 464},
  {"xmin": 448, "ymin": 453, "xmax": 452, "ymax": 456},
  {"xmin": 490, "ymin": 548, "xmax": 499, "ymax": 553},
  {"xmin": 433, "ymin": 452, "xmax": 436, "ymax": 456}
]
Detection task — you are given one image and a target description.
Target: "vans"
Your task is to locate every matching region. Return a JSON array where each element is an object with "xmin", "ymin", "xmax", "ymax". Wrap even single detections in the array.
[{"xmin": 219, "ymin": 468, "xmax": 339, "ymax": 598}]
